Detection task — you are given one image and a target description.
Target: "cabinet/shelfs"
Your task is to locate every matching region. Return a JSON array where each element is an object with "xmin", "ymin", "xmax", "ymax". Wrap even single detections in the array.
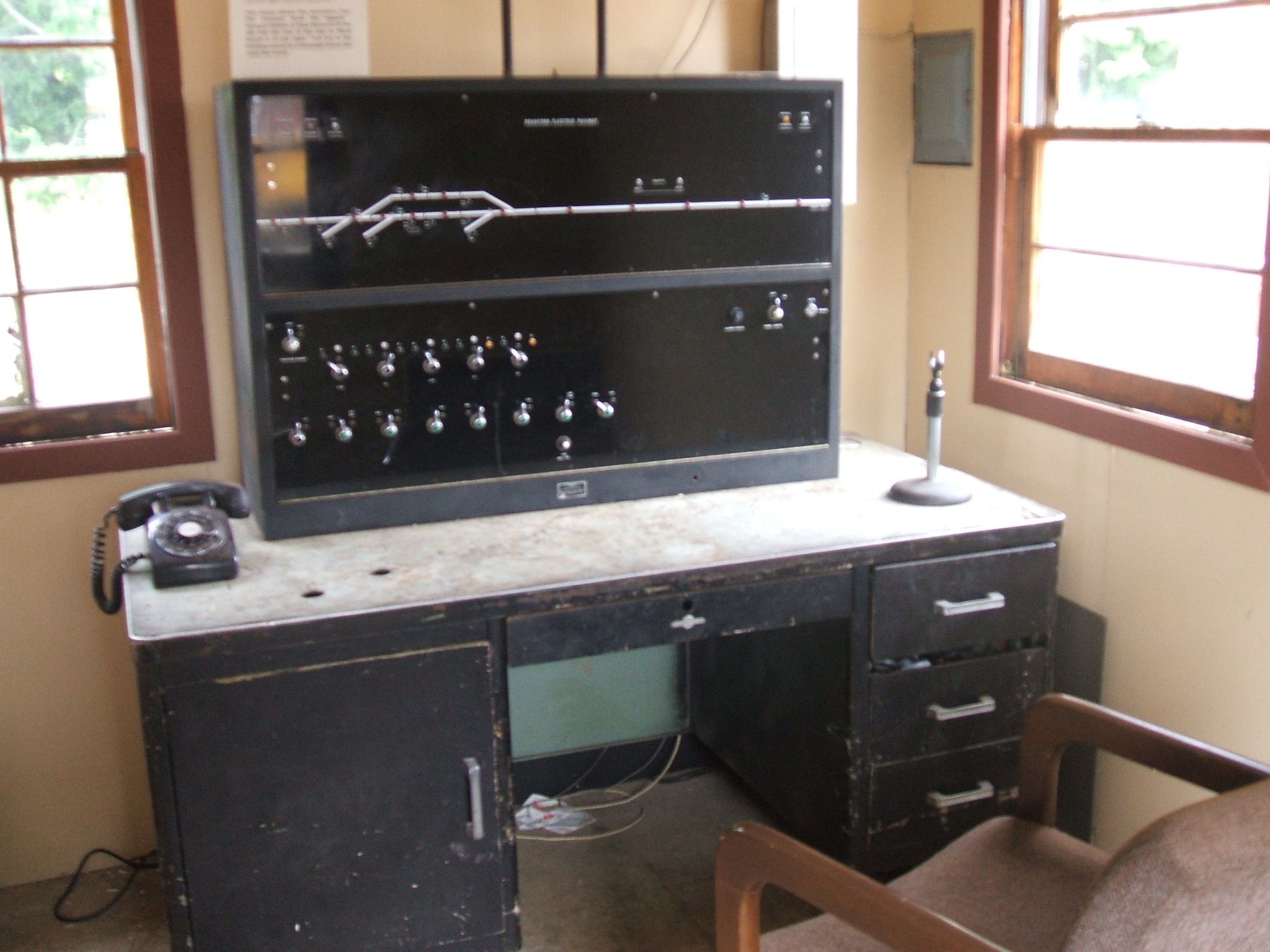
[{"xmin": 116, "ymin": 439, "xmax": 1065, "ymax": 952}]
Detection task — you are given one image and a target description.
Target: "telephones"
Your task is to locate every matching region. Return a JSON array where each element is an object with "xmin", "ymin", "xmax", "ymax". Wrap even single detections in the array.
[{"xmin": 116, "ymin": 479, "xmax": 251, "ymax": 589}]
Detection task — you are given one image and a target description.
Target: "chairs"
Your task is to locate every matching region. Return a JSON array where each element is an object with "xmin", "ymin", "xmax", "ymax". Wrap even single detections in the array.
[{"xmin": 716, "ymin": 690, "xmax": 1270, "ymax": 952}]
